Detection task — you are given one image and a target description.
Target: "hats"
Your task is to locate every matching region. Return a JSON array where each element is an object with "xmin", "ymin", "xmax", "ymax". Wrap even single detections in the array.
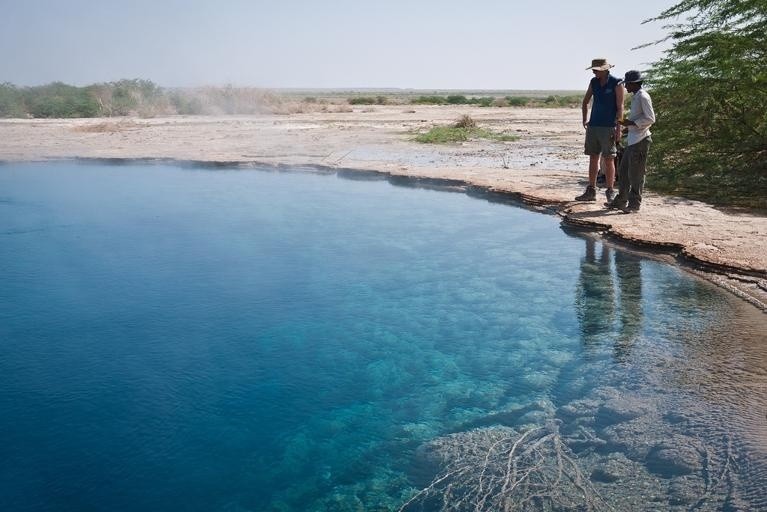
[
  {"xmin": 585, "ymin": 58, "xmax": 615, "ymax": 71},
  {"xmin": 617, "ymin": 70, "xmax": 644, "ymax": 85}
]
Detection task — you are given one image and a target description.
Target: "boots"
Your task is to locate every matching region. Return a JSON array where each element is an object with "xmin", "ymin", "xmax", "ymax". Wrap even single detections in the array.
[
  {"xmin": 605, "ymin": 189, "xmax": 615, "ymax": 202},
  {"xmin": 575, "ymin": 186, "xmax": 597, "ymax": 201}
]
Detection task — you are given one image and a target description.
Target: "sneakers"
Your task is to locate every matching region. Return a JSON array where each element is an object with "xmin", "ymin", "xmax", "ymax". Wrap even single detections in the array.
[
  {"xmin": 604, "ymin": 195, "xmax": 627, "ymax": 209},
  {"xmin": 623, "ymin": 203, "xmax": 641, "ymax": 213}
]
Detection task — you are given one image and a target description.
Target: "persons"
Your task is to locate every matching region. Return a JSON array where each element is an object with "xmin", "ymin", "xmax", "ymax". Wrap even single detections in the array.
[
  {"xmin": 576, "ymin": 236, "xmax": 616, "ymax": 363},
  {"xmin": 575, "ymin": 56, "xmax": 624, "ymax": 207},
  {"xmin": 604, "ymin": 69, "xmax": 656, "ymax": 213},
  {"xmin": 612, "ymin": 249, "xmax": 645, "ymax": 364}
]
